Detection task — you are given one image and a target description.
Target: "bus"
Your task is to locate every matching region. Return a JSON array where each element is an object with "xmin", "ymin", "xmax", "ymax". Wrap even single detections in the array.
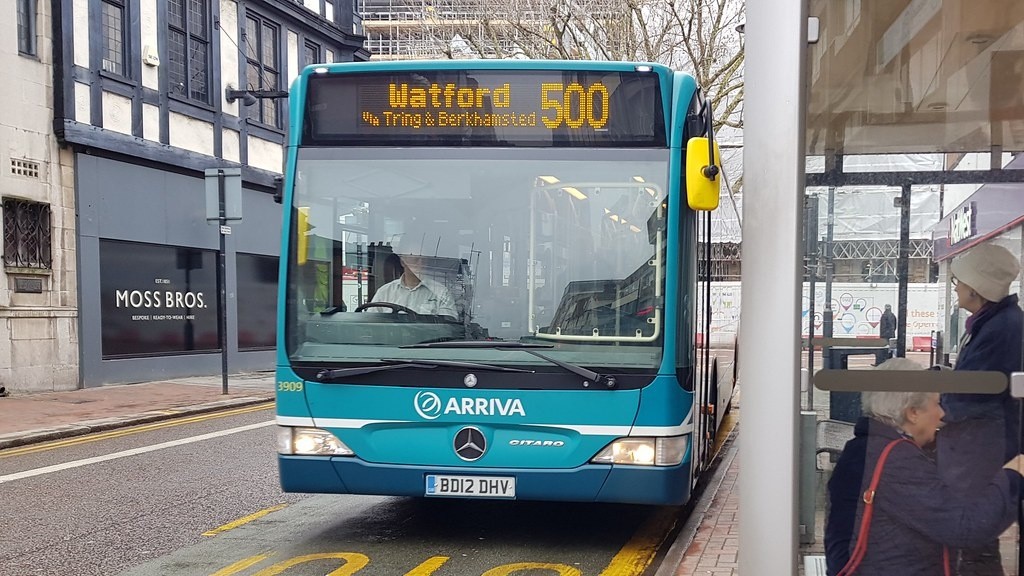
[{"xmin": 274, "ymin": 60, "xmax": 743, "ymax": 500}]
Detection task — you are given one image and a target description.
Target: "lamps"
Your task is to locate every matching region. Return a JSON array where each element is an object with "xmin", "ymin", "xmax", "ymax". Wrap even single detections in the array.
[{"xmin": 244, "ymin": 82, "xmax": 256, "ymax": 106}]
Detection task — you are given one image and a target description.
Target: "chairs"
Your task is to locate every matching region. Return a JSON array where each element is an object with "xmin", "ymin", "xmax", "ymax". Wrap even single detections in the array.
[{"xmin": 387, "ymin": 224, "xmax": 459, "ymax": 286}]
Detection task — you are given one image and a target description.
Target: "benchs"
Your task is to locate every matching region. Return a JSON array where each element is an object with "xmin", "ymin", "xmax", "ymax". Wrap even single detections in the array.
[{"xmin": 818, "ymin": 419, "xmax": 858, "ymax": 466}]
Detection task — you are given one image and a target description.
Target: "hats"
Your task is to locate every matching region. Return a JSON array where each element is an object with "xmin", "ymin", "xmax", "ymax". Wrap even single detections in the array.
[{"xmin": 950, "ymin": 242, "xmax": 1020, "ymax": 302}]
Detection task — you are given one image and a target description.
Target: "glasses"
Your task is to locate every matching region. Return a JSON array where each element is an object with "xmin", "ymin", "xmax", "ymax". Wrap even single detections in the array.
[{"xmin": 951, "ymin": 276, "xmax": 960, "ymax": 286}]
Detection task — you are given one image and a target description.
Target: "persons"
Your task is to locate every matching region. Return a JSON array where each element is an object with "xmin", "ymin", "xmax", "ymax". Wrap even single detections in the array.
[
  {"xmin": 519, "ymin": 229, "xmax": 626, "ymax": 307},
  {"xmin": 824, "ymin": 358, "xmax": 1024, "ymax": 576},
  {"xmin": 937, "ymin": 245, "xmax": 1024, "ymax": 576},
  {"xmin": 871, "ymin": 304, "xmax": 896, "ymax": 366},
  {"xmin": 366, "ymin": 236, "xmax": 458, "ymax": 321}
]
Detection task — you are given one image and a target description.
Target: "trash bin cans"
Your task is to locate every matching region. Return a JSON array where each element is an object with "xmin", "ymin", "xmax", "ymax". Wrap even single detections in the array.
[{"xmin": 829, "ymin": 347, "xmax": 893, "ymax": 423}]
